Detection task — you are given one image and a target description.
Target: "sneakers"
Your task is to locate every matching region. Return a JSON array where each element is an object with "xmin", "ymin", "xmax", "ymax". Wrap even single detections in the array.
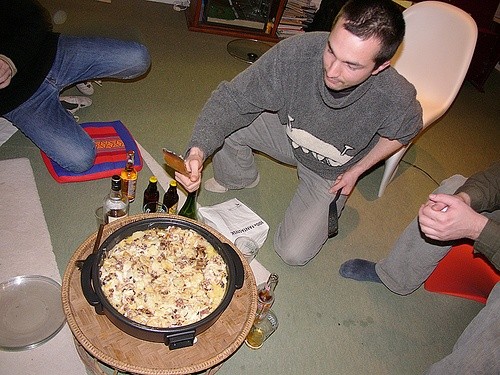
[
  {"xmin": 76, "ymin": 79, "xmax": 102, "ymax": 95},
  {"xmin": 59, "ymin": 96, "xmax": 92, "ymax": 123}
]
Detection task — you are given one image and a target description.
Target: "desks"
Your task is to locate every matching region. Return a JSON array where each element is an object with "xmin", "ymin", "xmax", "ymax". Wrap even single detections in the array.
[{"xmin": 62, "ymin": 212, "xmax": 258, "ymax": 375}]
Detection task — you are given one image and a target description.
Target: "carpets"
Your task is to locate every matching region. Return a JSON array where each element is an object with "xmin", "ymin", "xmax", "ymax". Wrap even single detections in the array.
[{"xmin": 1, "ymin": 158, "xmax": 88, "ymax": 375}]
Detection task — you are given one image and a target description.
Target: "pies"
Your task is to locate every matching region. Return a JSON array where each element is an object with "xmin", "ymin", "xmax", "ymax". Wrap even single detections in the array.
[{"xmin": 98, "ymin": 226, "xmax": 227, "ymax": 328}]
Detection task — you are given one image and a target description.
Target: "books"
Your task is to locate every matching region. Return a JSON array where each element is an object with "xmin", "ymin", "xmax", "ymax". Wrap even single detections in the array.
[{"xmin": 275, "ymin": 0, "xmax": 317, "ymax": 38}]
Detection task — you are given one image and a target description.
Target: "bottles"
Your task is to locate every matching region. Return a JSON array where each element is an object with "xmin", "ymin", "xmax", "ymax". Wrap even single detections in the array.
[
  {"xmin": 161, "ymin": 181, "xmax": 180, "ymax": 215},
  {"xmin": 121, "ymin": 150, "xmax": 138, "ymax": 203},
  {"xmin": 252, "ymin": 273, "xmax": 278, "ymax": 327},
  {"xmin": 102, "ymin": 174, "xmax": 129, "ymax": 224},
  {"xmin": 142, "ymin": 175, "xmax": 160, "ymax": 213},
  {"xmin": 178, "ymin": 189, "xmax": 198, "ymax": 222}
]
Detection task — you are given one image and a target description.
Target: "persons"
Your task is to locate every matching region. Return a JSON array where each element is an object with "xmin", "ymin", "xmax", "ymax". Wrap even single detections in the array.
[
  {"xmin": 175, "ymin": 0, "xmax": 423, "ymax": 267},
  {"xmin": 0, "ymin": 0, "xmax": 151, "ymax": 173},
  {"xmin": 339, "ymin": 162, "xmax": 500, "ymax": 375}
]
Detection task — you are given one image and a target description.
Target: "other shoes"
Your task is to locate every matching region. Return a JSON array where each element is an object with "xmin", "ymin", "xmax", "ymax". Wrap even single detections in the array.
[{"xmin": 204, "ymin": 169, "xmax": 260, "ymax": 193}]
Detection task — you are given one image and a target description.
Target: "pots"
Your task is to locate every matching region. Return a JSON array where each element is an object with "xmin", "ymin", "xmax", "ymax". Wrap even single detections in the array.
[{"xmin": 79, "ymin": 216, "xmax": 245, "ymax": 352}]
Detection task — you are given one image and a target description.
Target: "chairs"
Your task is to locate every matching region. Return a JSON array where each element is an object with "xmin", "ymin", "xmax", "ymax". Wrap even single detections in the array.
[{"xmin": 377, "ymin": 0, "xmax": 478, "ymax": 198}]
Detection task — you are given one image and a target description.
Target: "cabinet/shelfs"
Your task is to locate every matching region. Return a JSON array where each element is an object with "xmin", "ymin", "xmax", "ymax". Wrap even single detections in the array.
[{"xmin": 184, "ymin": 0, "xmax": 289, "ymax": 43}]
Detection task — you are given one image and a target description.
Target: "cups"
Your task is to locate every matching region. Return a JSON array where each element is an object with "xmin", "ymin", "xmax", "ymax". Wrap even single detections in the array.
[
  {"xmin": 94, "ymin": 207, "xmax": 104, "ymax": 230},
  {"xmin": 244, "ymin": 306, "xmax": 280, "ymax": 350},
  {"xmin": 143, "ymin": 202, "xmax": 168, "ymax": 213},
  {"xmin": 233, "ymin": 236, "xmax": 259, "ymax": 265}
]
